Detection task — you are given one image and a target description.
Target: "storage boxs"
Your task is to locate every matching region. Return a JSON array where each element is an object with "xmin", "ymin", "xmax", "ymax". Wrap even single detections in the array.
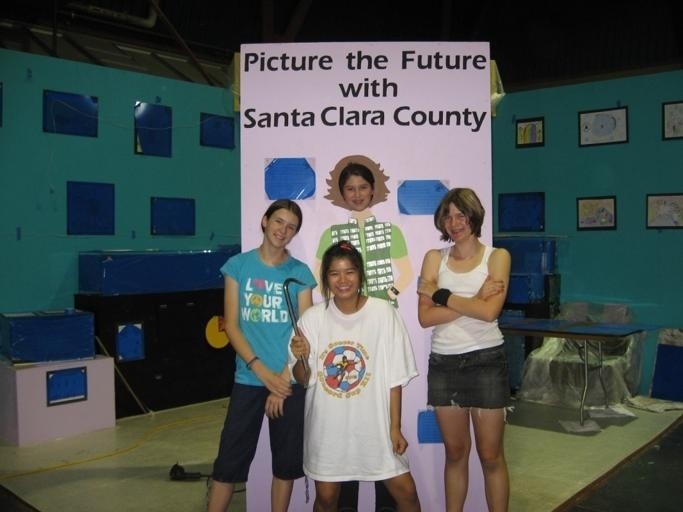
[
  {"xmin": 0, "ymin": 309, "xmax": 96, "ymax": 365},
  {"xmin": 0, "ymin": 354, "xmax": 116, "ymax": 448}
]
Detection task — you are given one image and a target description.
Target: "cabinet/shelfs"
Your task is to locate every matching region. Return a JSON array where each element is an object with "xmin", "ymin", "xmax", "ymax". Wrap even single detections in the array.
[{"xmin": 73, "ymin": 288, "xmax": 236, "ymax": 420}]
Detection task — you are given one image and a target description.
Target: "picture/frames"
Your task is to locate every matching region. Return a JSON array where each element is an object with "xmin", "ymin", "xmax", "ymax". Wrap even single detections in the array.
[
  {"xmin": 577, "ymin": 196, "xmax": 617, "ymax": 231},
  {"xmin": 646, "ymin": 193, "xmax": 683, "ymax": 230},
  {"xmin": 578, "ymin": 105, "xmax": 629, "ymax": 147},
  {"xmin": 661, "ymin": 101, "xmax": 683, "ymax": 140},
  {"xmin": 516, "ymin": 116, "xmax": 545, "ymax": 149}
]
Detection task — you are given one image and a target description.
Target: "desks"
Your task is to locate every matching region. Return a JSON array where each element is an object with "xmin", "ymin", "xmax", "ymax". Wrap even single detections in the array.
[{"xmin": 498, "ymin": 318, "xmax": 644, "ymax": 428}]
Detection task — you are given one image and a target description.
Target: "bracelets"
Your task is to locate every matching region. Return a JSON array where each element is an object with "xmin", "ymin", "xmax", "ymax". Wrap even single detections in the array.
[
  {"xmin": 242, "ymin": 356, "xmax": 259, "ymax": 369},
  {"xmin": 430, "ymin": 287, "xmax": 451, "ymax": 307}
]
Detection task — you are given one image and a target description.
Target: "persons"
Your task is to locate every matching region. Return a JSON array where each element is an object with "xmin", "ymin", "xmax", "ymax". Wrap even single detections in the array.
[
  {"xmin": 414, "ymin": 186, "xmax": 513, "ymax": 510},
  {"xmin": 337, "ymin": 163, "xmax": 374, "ymax": 213},
  {"xmin": 284, "ymin": 238, "xmax": 425, "ymax": 512},
  {"xmin": 201, "ymin": 196, "xmax": 320, "ymax": 511}
]
